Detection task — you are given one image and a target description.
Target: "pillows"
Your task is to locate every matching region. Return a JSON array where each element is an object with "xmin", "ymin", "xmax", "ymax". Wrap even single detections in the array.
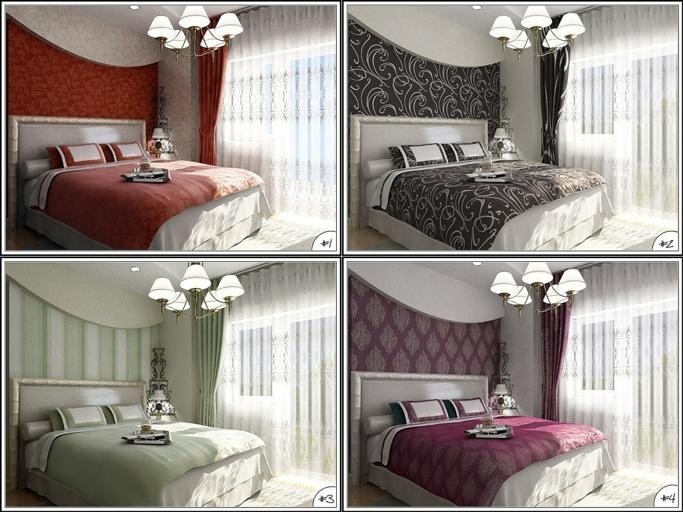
[
  {"xmin": 101, "ymin": 406, "xmax": 117, "ymax": 429},
  {"xmin": 45, "ymin": 409, "xmax": 62, "ymax": 432},
  {"xmin": 111, "ymin": 405, "xmax": 147, "ymax": 422},
  {"xmin": 442, "ymin": 399, "xmax": 458, "ymax": 422},
  {"xmin": 443, "ymin": 142, "xmax": 490, "ymax": 165},
  {"xmin": 105, "ymin": 139, "xmax": 149, "ymax": 162},
  {"xmin": 392, "ymin": 143, "xmax": 449, "ymax": 169},
  {"xmin": 56, "ymin": 402, "xmax": 106, "ymax": 430},
  {"xmin": 47, "ymin": 141, "xmax": 106, "ymax": 168},
  {"xmin": 451, "ymin": 396, "xmax": 487, "ymax": 420},
  {"xmin": 401, "ymin": 398, "xmax": 449, "ymax": 426},
  {"xmin": 390, "ymin": 402, "xmax": 408, "ymax": 427}
]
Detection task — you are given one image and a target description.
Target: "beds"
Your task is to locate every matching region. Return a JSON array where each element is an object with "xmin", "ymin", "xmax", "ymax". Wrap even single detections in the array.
[
  {"xmin": 9, "ymin": 376, "xmax": 276, "ymax": 509},
  {"xmin": 349, "ymin": 370, "xmax": 616, "ymax": 508},
  {"xmin": 4, "ymin": 115, "xmax": 271, "ymax": 252},
  {"xmin": 346, "ymin": 115, "xmax": 619, "ymax": 254}
]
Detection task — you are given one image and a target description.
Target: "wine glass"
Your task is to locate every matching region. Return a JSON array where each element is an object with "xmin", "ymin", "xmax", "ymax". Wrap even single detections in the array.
[
  {"xmin": 141, "ymin": 419, "xmax": 152, "ymax": 440},
  {"xmin": 483, "ymin": 414, "xmax": 494, "ymax": 435},
  {"xmin": 140, "ymin": 156, "xmax": 151, "ymax": 179},
  {"xmin": 482, "ymin": 157, "xmax": 492, "ymax": 178}
]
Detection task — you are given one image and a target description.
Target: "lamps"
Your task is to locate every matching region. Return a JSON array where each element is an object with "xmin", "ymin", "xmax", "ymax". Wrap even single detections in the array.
[
  {"xmin": 489, "ymin": 6, "xmax": 585, "ymax": 62},
  {"xmin": 151, "ymin": 390, "xmax": 167, "ymax": 420},
  {"xmin": 494, "ymin": 383, "xmax": 508, "ymax": 408},
  {"xmin": 147, "ymin": 6, "xmax": 243, "ymax": 64},
  {"xmin": 490, "ymin": 262, "xmax": 587, "ymax": 320},
  {"xmin": 493, "ymin": 128, "xmax": 507, "ymax": 155},
  {"xmin": 151, "ymin": 127, "xmax": 166, "ymax": 152},
  {"xmin": 145, "ymin": 262, "xmax": 246, "ymax": 325}
]
